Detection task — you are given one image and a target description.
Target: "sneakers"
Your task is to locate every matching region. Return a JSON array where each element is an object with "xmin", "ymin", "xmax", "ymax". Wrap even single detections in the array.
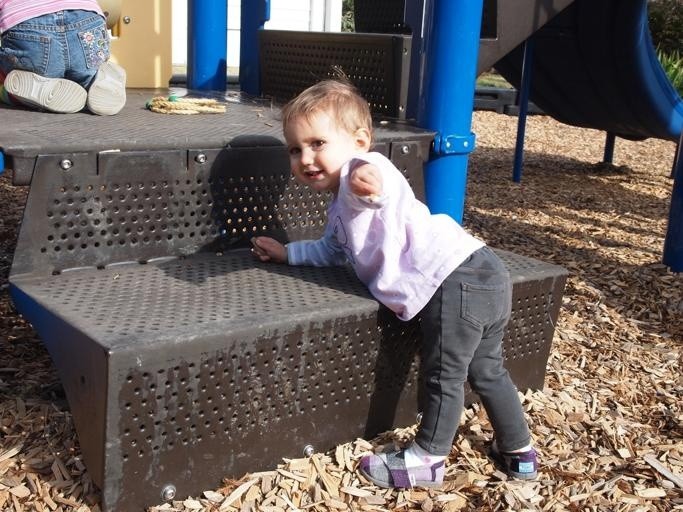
[
  {"xmin": 81, "ymin": 57, "xmax": 130, "ymax": 115},
  {"xmin": 358, "ymin": 444, "xmax": 446, "ymax": 492},
  {"xmin": 489, "ymin": 427, "xmax": 540, "ymax": 481},
  {"xmin": 1, "ymin": 68, "xmax": 86, "ymax": 116}
]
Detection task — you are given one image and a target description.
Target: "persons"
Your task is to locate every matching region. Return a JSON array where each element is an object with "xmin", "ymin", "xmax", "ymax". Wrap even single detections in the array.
[
  {"xmin": 1, "ymin": 1, "xmax": 129, "ymax": 116},
  {"xmin": 249, "ymin": 79, "xmax": 539, "ymax": 489}
]
